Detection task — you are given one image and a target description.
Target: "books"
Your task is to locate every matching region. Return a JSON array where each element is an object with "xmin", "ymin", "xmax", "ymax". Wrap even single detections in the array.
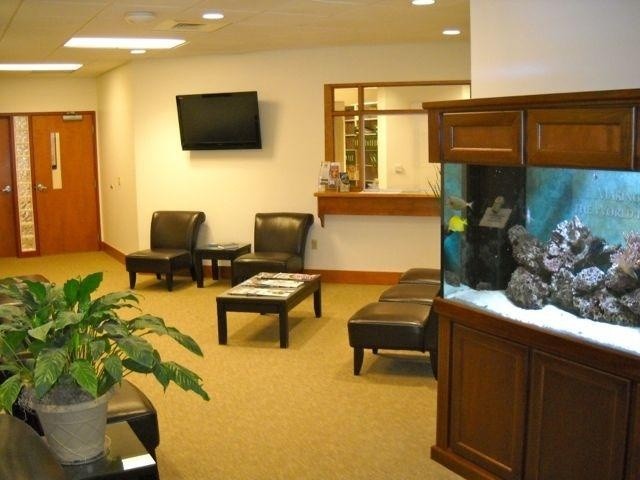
[{"xmin": 227, "ymin": 272, "xmax": 316, "ymax": 297}]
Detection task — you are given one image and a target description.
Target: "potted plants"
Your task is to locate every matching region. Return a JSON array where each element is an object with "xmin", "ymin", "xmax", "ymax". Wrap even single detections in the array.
[{"xmin": 1, "ymin": 268, "xmax": 210, "ymax": 463}]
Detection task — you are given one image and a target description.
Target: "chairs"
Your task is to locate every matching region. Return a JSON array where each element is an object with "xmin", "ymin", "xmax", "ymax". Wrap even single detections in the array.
[
  {"xmin": 233, "ymin": 213, "xmax": 314, "ymax": 287},
  {"xmin": 125, "ymin": 210, "xmax": 205, "ymax": 292},
  {"xmin": 349, "ymin": 269, "xmax": 440, "ymax": 380}
]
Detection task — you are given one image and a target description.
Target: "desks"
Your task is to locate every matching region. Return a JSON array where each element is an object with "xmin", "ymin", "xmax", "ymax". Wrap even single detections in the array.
[
  {"xmin": 39, "ymin": 421, "xmax": 157, "ymax": 480},
  {"xmin": 196, "ymin": 244, "xmax": 250, "ymax": 289}
]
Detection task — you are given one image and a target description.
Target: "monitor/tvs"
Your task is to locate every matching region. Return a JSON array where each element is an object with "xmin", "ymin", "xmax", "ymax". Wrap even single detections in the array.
[{"xmin": 176, "ymin": 90, "xmax": 262, "ymax": 150}]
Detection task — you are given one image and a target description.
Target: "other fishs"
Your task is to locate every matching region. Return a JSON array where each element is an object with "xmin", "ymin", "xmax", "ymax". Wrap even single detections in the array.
[
  {"xmin": 447, "ymin": 195, "xmax": 473, "ymax": 210},
  {"xmin": 448, "ymin": 215, "xmax": 468, "ymax": 232}
]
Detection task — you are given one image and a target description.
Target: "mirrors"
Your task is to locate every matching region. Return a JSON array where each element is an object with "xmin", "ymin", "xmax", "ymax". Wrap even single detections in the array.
[{"xmin": 324, "ymin": 80, "xmax": 473, "ymax": 192}]
